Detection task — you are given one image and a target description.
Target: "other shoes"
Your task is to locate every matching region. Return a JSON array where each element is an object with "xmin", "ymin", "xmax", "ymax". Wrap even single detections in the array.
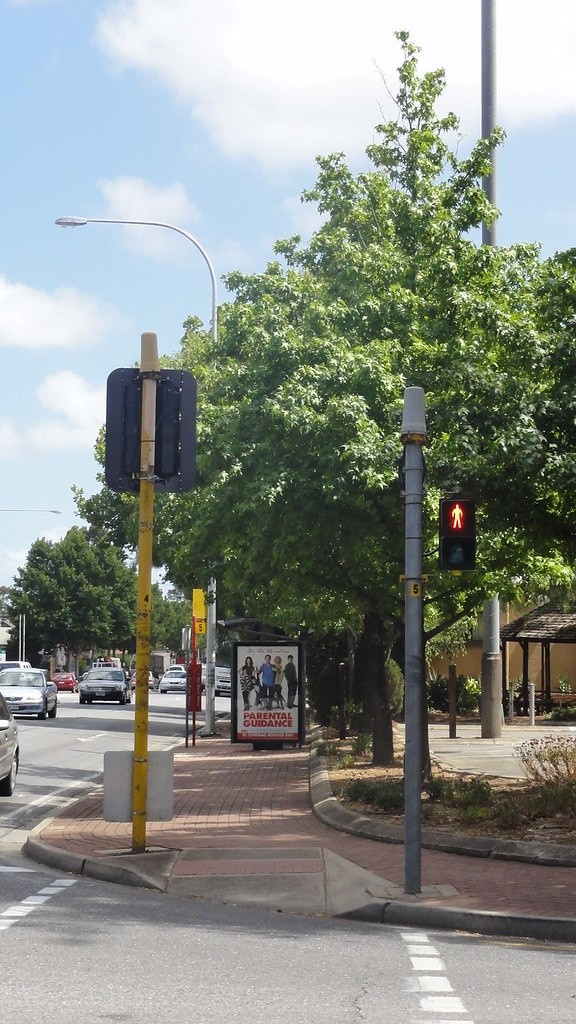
[
  {"xmin": 267, "ymin": 705, "xmax": 272, "ymax": 711},
  {"xmin": 276, "ymin": 704, "xmax": 279, "ymax": 708},
  {"xmin": 281, "ymin": 705, "xmax": 284, "ymax": 710},
  {"xmin": 264, "ymin": 702, "xmax": 269, "ymax": 708},
  {"xmin": 292, "ymin": 704, "xmax": 297, "ymax": 707},
  {"xmin": 287, "ymin": 704, "xmax": 292, "ymax": 709},
  {"xmin": 244, "ymin": 705, "xmax": 249, "ymax": 711}
]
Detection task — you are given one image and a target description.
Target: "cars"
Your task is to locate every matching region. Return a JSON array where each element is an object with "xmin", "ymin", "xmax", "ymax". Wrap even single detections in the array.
[
  {"xmin": 0, "ymin": 668, "xmax": 57, "ymax": 720},
  {"xmin": 78, "ymin": 667, "xmax": 132, "ymax": 705},
  {"xmin": 51, "ymin": 673, "xmax": 79, "ymax": 693},
  {"xmin": 0, "ymin": 692, "xmax": 20, "ymax": 797},
  {"xmin": 159, "ymin": 670, "xmax": 187, "ymax": 694},
  {"xmin": 131, "ymin": 671, "xmax": 160, "ymax": 691}
]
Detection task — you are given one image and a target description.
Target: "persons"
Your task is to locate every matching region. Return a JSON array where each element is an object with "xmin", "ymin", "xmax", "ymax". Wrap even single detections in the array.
[{"xmin": 238, "ymin": 654, "xmax": 298, "ymax": 711}]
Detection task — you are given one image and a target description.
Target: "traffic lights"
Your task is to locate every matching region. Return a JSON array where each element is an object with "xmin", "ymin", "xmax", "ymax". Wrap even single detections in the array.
[{"xmin": 439, "ymin": 499, "xmax": 476, "ymax": 570}]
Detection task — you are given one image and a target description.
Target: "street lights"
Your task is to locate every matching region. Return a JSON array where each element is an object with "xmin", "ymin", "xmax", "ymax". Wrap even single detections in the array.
[
  {"xmin": 55, "ymin": 216, "xmax": 218, "ymax": 737},
  {"xmin": 0, "ymin": 510, "xmax": 62, "ymax": 514}
]
